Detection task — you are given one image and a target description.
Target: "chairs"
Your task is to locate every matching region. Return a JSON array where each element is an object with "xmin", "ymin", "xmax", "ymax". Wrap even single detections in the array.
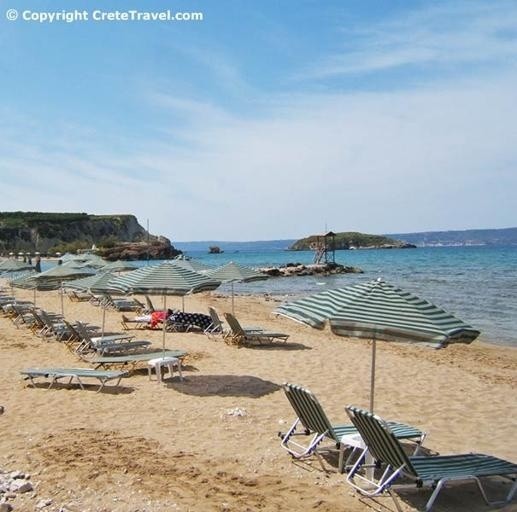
[
  {"xmin": 277, "ymin": 383, "xmax": 517, "ymax": 512},
  {"xmin": 1, "ymin": 288, "xmax": 289, "ymax": 394}
]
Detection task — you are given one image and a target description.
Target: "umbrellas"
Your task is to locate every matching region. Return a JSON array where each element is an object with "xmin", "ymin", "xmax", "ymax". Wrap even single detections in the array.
[
  {"xmin": 271, "ymin": 277, "xmax": 480, "ymax": 413},
  {"xmin": 0, "ymin": 252, "xmax": 270, "ymax": 359}
]
